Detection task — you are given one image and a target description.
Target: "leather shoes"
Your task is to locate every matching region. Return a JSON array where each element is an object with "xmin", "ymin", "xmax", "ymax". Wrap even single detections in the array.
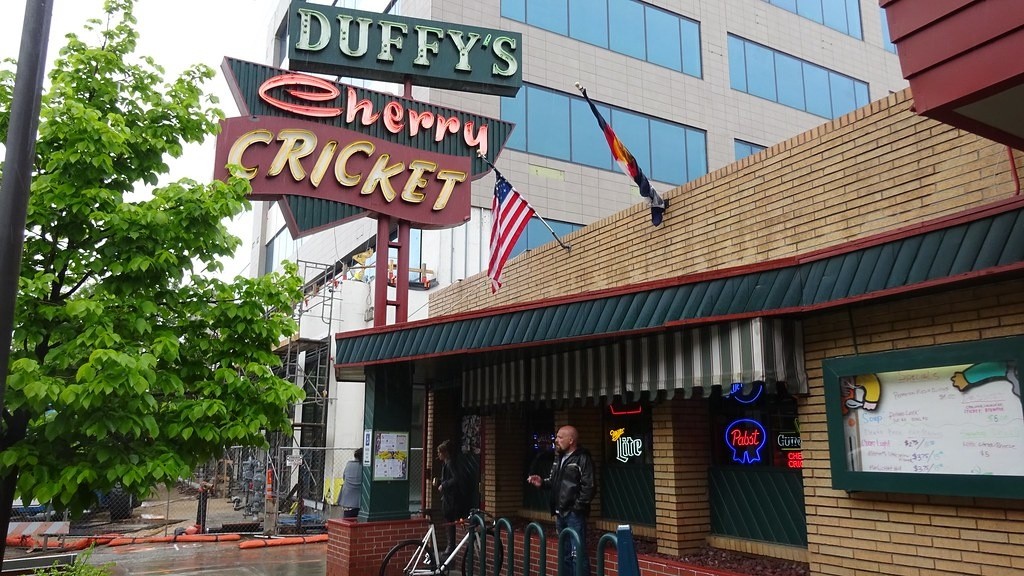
[{"xmin": 441, "ymin": 546, "xmax": 455, "ymax": 555}]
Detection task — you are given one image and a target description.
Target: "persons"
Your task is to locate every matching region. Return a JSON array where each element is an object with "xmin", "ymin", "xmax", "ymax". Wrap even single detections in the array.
[
  {"xmin": 337, "ymin": 448, "xmax": 364, "ymax": 517},
  {"xmin": 527, "ymin": 425, "xmax": 595, "ymax": 576},
  {"xmin": 432, "ymin": 439, "xmax": 468, "ymax": 555}
]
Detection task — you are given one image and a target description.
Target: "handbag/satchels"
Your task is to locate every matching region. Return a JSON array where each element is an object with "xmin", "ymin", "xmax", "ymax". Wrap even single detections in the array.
[{"xmin": 443, "ymin": 460, "xmax": 478, "ymax": 511}]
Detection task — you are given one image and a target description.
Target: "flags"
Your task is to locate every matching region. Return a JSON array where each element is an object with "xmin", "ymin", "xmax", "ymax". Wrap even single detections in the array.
[
  {"xmin": 582, "ymin": 90, "xmax": 666, "ymax": 227},
  {"xmin": 487, "ymin": 169, "xmax": 537, "ymax": 294}
]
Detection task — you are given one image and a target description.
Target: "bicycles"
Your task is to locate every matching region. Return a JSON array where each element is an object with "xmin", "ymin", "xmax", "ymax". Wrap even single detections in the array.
[{"xmin": 379, "ymin": 508, "xmax": 504, "ymax": 576}]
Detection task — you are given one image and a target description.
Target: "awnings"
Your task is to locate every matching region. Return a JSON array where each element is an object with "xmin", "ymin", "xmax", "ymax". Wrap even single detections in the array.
[{"xmin": 459, "ymin": 317, "xmax": 808, "ymax": 414}]
